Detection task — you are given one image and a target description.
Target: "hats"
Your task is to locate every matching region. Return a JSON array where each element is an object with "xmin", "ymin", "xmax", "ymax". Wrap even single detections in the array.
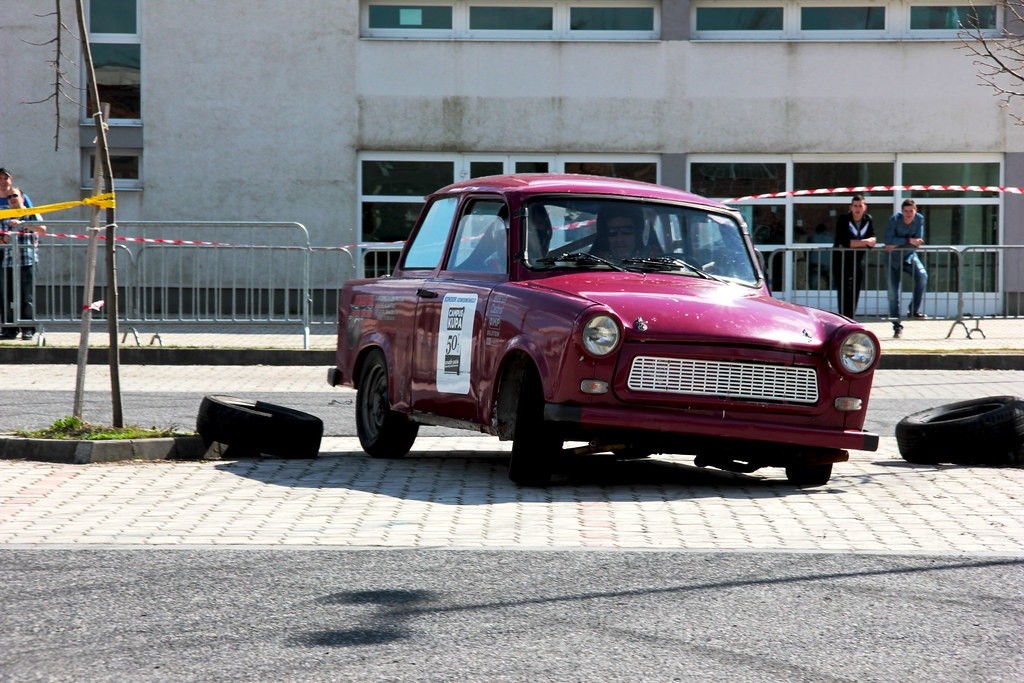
[{"xmin": 0, "ymin": 167, "xmax": 13, "ymax": 177}]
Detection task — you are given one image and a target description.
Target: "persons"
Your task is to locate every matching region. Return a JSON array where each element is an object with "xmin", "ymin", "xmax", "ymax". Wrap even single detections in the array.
[
  {"xmin": 885, "ymin": 199, "xmax": 929, "ymax": 338},
  {"xmin": 0, "ymin": 168, "xmax": 47, "ymax": 339},
  {"xmin": 477, "ymin": 202, "xmax": 552, "ymax": 273},
  {"xmin": 834, "ymin": 195, "xmax": 876, "ymax": 319},
  {"xmin": 605, "ymin": 211, "xmax": 641, "ymax": 257},
  {"xmin": 793, "ymin": 224, "xmax": 836, "ymax": 291}
]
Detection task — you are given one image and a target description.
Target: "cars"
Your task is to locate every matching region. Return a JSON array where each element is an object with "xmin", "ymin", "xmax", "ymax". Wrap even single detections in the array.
[{"xmin": 327, "ymin": 172, "xmax": 879, "ymax": 489}]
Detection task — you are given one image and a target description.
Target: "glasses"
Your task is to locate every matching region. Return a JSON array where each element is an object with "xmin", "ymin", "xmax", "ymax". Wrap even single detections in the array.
[
  {"xmin": 7, "ymin": 193, "xmax": 18, "ymax": 199},
  {"xmin": 606, "ymin": 225, "xmax": 638, "ymax": 237}
]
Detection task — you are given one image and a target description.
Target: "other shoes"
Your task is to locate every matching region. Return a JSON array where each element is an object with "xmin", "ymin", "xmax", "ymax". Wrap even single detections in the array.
[
  {"xmin": 893, "ymin": 324, "xmax": 904, "ymax": 338},
  {"xmin": 907, "ymin": 311, "xmax": 926, "ymax": 319},
  {"xmin": 22, "ymin": 331, "xmax": 32, "ymax": 340},
  {"xmin": 0, "ymin": 329, "xmax": 17, "ymax": 339}
]
